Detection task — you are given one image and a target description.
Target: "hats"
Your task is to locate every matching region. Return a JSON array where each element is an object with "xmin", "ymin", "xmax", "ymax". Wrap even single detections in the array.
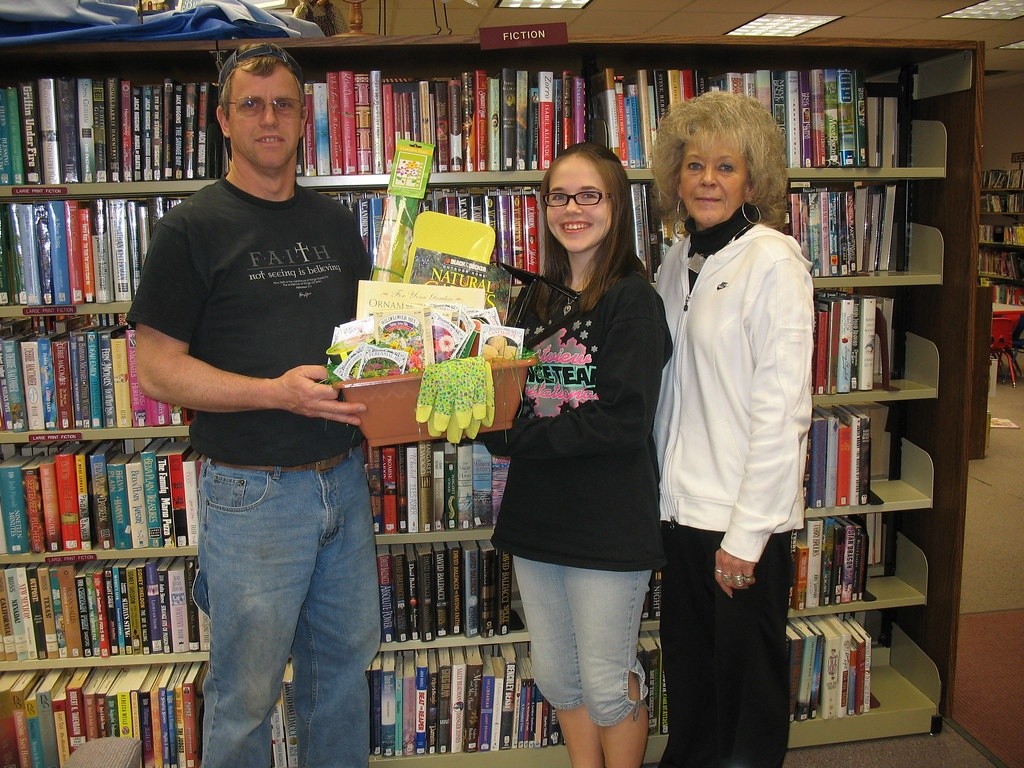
[{"xmin": 218, "ymin": 42, "xmax": 305, "ymax": 98}]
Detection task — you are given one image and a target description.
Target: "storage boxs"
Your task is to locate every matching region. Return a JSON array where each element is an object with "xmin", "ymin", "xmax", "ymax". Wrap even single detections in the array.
[{"xmin": 331, "ymin": 337, "xmax": 538, "ymax": 449}]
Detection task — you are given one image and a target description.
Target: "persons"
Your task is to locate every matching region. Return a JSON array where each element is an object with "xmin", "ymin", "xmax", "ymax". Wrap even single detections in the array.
[{"xmin": 119, "ymin": 41, "xmax": 386, "ymax": 766}]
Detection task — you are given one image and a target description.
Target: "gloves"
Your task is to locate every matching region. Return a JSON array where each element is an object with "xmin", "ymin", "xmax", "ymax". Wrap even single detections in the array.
[{"xmin": 414, "ymin": 356, "xmax": 496, "ymax": 445}]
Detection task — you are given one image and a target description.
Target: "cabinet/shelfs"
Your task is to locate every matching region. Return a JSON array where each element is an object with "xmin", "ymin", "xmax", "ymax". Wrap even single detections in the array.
[
  {"xmin": 975, "ymin": 165, "xmax": 1024, "ymax": 307},
  {"xmin": 0, "ymin": 35, "xmax": 985, "ymax": 765}
]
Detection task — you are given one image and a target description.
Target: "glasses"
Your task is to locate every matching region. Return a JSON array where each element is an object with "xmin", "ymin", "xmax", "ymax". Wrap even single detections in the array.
[
  {"xmin": 543, "ymin": 191, "xmax": 612, "ymax": 208},
  {"xmin": 228, "ymin": 97, "xmax": 305, "ymax": 117}
]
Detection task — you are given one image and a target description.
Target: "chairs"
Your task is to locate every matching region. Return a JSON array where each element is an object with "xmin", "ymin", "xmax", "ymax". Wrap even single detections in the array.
[{"xmin": 991, "ymin": 317, "xmax": 1018, "ymax": 389}]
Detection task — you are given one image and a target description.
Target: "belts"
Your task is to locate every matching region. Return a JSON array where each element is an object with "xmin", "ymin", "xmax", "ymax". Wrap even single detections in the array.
[{"xmin": 200, "ymin": 451, "xmax": 351, "ymax": 472}]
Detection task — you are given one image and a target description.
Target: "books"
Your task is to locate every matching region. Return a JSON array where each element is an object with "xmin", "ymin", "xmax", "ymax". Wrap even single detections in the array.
[
  {"xmin": 1, "ymin": 70, "xmax": 904, "ymax": 765},
  {"xmin": 976, "ymin": 166, "xmax": 1023, "ymax": 308}
]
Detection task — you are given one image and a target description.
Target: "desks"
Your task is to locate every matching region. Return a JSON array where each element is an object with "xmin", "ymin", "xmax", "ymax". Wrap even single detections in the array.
[{"xmin": 989, "ymin": 299, "xmax": 1024, "ymax": 320}]
